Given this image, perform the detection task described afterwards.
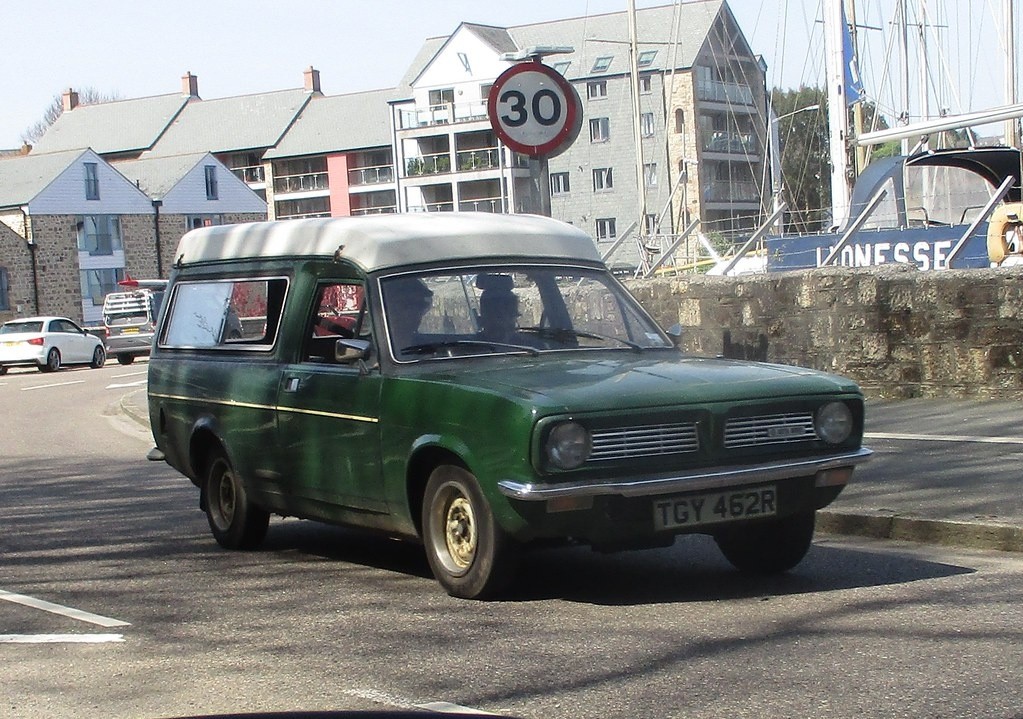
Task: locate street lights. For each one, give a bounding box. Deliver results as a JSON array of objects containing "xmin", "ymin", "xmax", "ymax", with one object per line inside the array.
[{"xmin": 769, "ymin": 105, "xmax": 819, "ymax": 190}]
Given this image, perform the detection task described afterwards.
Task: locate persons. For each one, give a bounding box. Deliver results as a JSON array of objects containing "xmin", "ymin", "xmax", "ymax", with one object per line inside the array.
[
  {"xmin": 382, "ymin": 278, "xmax": 433, "ymax": 348},
  {"xmin": 468, "ymin": 289, "xmax": 550, "ymax": 350}
]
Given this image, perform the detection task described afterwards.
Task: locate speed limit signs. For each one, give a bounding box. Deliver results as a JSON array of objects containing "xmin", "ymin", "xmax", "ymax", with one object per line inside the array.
[{"xmin": 488, "ymin": 62, "xmax": 576, "ymax": 156}]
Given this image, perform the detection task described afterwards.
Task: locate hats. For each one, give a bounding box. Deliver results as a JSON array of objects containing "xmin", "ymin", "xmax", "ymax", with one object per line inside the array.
[{"xmin": 385, "ymin": 277, "xmax": 433, "ymax": 298}]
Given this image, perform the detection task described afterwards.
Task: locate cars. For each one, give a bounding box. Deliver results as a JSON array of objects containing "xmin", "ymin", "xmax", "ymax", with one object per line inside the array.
[
  {"xmin": 148, "ymin": 211, "xmax": 874, "ymax": 601},
  {"xmin": 0, "ymin": 316, "xmax": 107, "ymax": 377}
]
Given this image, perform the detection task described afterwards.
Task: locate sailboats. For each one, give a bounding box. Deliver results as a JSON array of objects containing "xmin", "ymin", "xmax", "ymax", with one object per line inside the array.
[{"xmin": 584, "ymin": 0, "xmax": 1022, "ymax": 278}]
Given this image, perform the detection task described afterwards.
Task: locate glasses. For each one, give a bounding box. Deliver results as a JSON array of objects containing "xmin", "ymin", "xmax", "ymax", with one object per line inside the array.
[
  {"xmin": 490, "ymin": 312, "xmax": 522, "ymax": 320},
  {"xmin": 392, "ymin": 301, "xmax": 431, "ymax": 315}
]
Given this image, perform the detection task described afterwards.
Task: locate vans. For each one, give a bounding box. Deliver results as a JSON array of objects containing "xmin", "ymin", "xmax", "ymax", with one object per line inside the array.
[{"xmin": 102, "ymin": 289, "xmax": 244, "ymax": 365}]
